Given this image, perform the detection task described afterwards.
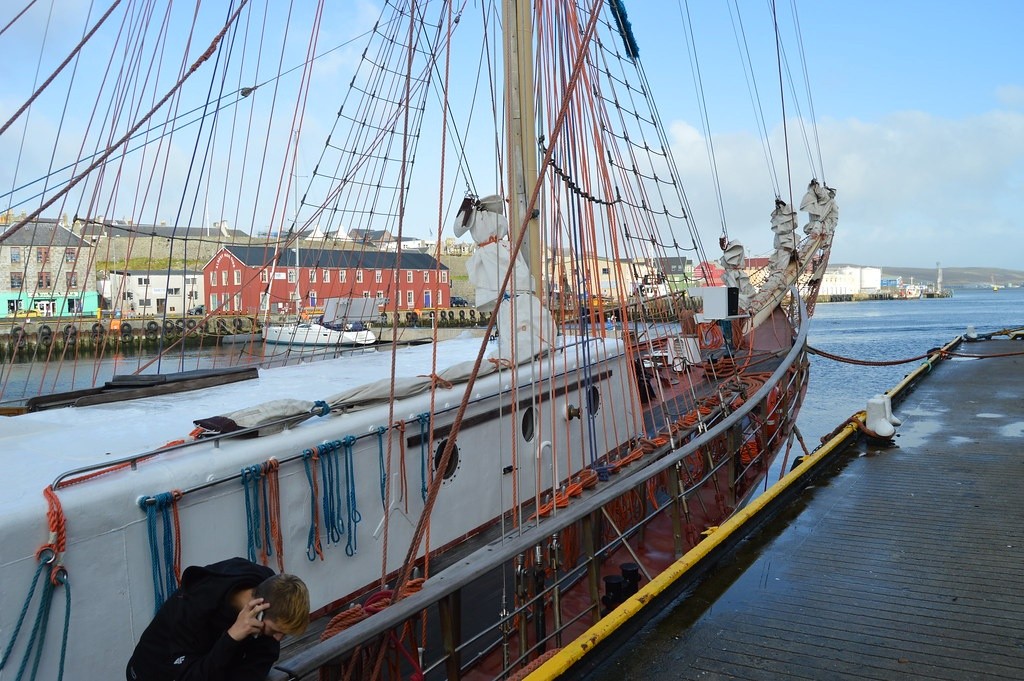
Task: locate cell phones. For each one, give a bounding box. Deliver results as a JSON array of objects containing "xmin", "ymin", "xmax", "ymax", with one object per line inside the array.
[{"xmin": 251, "ymin": 604, "xmax": 264, "ymax": 639}]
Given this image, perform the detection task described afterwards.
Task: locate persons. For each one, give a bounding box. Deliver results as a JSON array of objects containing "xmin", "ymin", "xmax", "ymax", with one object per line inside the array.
[{"xmin": 126, "ymin": 557, "xmax": 310, "ymax": 681}]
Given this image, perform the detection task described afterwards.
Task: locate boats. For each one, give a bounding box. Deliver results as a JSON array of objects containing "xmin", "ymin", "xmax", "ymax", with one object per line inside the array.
[
  {"xmin": 220, "ymin": 332, "xmax": 262, "ymax": 343},
  {"xmin": 629, "ymin": 240, "xmax": 673, "ymax": 321}
]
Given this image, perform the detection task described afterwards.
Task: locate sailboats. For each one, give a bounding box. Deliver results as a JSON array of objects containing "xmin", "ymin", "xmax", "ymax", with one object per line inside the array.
[
  {"xmin": 261, "ymin": 129, "xmax": 376, "ymax": 345},
  {"xmin": 0, "ymin": 1, "xmax": 838, "ymax": 681}
]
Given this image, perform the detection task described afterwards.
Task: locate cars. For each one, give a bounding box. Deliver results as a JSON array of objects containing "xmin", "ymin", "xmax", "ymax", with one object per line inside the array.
[
  {"xmin": 7, "ymin": 309, "xmax": 42, "ymax": 317},
  {"xmin": 450, "ymin": 297, "xmax": 468, "ymax": 307},
  {"xmin": 188, "ymin": 305, "xmax": 204, "ymax": 315}
]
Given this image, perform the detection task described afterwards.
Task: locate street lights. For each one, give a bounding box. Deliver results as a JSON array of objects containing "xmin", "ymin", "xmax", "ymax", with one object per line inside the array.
[{"xmin": 113, "ymin": 235, "xmax": 120, "ymax": 278}]
